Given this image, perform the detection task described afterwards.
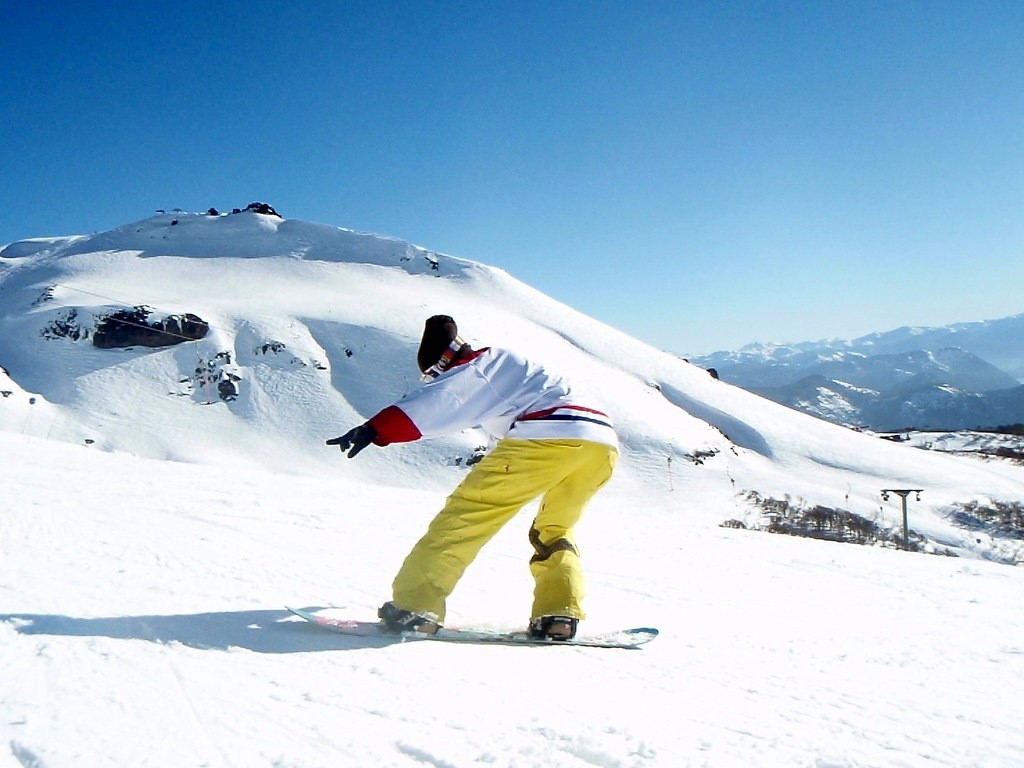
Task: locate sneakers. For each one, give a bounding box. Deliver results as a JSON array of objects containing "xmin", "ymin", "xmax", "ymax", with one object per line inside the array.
[
  {"xmin": 377, "ymin": 600, "xmax": 443, "ymax": 637},
  {"xmin": 526, "ymin": 614, "xmax": 579, "ymax": 640}
]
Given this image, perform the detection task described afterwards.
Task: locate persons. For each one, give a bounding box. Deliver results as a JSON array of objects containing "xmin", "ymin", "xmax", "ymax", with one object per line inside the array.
[{"xmin": 325, "ymin": 315, "xmax": 620, "ymax": 641}]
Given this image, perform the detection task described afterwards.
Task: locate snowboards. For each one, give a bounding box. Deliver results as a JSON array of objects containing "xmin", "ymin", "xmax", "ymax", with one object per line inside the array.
[{"xmin": 285, "ymin": 604, "xmax": 660, "ymax": 649}]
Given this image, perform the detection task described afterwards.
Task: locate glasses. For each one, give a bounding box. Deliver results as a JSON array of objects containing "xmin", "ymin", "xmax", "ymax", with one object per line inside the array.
[{"xmin": 419, "ymin": 364, "xmax": 445, "ymax": 383}]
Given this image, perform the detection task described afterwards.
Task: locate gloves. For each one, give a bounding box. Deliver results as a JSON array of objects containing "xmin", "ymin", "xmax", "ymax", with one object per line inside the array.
[{"xmin": 326, "ymin": 422, "xmax": 376, "ymax": 458}]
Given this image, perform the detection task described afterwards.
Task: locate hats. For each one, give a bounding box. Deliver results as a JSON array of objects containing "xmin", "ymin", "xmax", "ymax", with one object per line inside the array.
[{"xmin": 417, "ymin": 315, "xmax": 473, "ymax": 384}]
